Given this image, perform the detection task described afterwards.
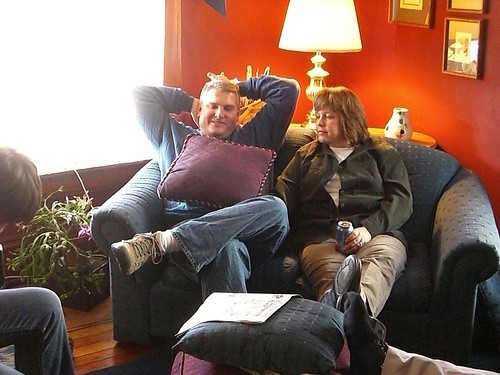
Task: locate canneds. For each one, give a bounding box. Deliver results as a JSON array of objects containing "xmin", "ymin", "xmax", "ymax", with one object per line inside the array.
[{"xmin": 335, "ymin": 221, "xmax": 354, "ymax": 254}]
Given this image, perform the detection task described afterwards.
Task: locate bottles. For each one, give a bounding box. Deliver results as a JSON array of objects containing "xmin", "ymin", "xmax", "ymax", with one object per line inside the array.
[{"xmin": 384, "ymin": 108, "xmax": 412, "ymax": 142}]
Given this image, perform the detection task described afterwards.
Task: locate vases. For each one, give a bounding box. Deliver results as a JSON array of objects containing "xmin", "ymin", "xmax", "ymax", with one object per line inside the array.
[{"xmin": 48, "ymin": 259, "xmax": 109, "ymax": 311}]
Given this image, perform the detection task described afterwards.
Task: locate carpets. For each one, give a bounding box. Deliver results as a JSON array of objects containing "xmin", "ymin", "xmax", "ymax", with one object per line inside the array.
[{"xmin": 82, "ymin": 342, "xmax": 176, "ymax": 375}]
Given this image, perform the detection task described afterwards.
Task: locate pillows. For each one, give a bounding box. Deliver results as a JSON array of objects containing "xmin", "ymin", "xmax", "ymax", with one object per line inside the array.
[
  {"xmin": 157, "ymin": 132, "xmax": 276, "ymax": 209},
  {"xmin": 170, "ymin": 298, "xmax": 346, "ymax": 375}
]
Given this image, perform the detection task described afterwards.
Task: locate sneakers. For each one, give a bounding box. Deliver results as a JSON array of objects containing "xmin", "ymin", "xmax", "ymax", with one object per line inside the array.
[{"xmin": 111, "ymin": 231, "xmax": 166, "ymax": 276}]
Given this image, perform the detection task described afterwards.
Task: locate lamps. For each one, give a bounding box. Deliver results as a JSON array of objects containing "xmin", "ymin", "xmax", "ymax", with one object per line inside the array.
[{"xmin": 277, "ymin": 0, "xmax": 363, "ymax": 129}]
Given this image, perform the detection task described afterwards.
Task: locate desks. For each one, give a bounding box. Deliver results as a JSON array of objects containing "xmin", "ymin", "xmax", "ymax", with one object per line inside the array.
[{"xmin": 288, "ymin": 122, "xmax": 438, "ymax": 148}]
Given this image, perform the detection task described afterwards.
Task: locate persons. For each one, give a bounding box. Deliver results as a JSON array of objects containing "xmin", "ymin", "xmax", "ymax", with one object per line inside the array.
[
  {"xmin": 111, "ymin": 75, "xmax": 301, "ymax": 303},
  {"xmin": 271, "ymin": 88, "xmax": 413, "ymax": 319},
  {"xmin": 0, "ymin": 148, "xmax": 75, "ymax": 375},
  {"xmin": 343, "ymin": 292, "xmax": 500, "ymax": 375}
]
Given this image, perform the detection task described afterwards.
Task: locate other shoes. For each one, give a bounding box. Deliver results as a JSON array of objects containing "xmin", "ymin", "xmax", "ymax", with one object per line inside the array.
[{"xmin": 344, "ymin": 292, "xmax": 389, "ymax": 375}]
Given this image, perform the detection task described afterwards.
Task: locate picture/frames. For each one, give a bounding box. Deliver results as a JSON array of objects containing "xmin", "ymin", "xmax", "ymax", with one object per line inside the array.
[
  {"xmin": 387, "ymin": 0, "xmax": 435, "ymax": 29},
  {"xmin": 441, "ymin": 17, "xmax": 485, "ymax": 79},
  {"xmin": 446, "ymin": 0, "xmax": 487, "ymax": 15}
]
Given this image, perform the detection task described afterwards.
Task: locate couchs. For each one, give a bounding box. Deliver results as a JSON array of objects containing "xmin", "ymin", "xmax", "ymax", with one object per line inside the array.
[{"xmin": 90, "ymin": 127, "xmax": 500, "ymax": 365}]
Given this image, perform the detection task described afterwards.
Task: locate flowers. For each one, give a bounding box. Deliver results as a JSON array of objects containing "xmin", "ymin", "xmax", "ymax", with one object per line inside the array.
[{"xmin": 6, "ymin": 184, "xmax": 106, "ymax": 300}]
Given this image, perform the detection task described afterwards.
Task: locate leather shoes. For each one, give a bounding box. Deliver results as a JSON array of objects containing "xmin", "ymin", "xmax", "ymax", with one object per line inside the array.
[{"xmin": 325, "ymin": 255, "xmax": 362, "ymax": 312}]
